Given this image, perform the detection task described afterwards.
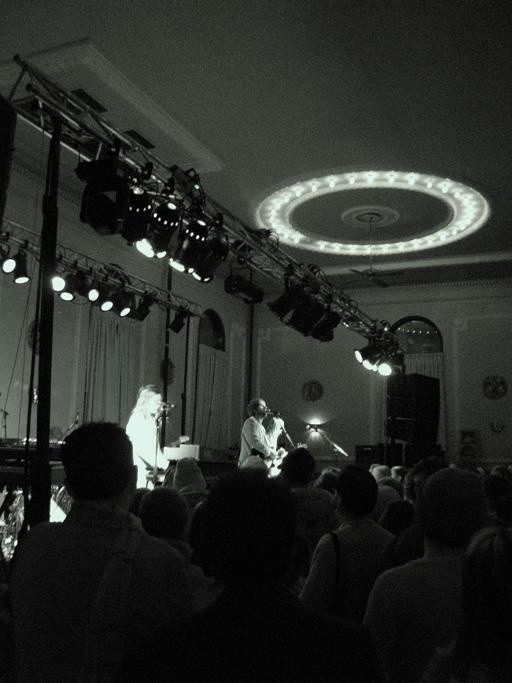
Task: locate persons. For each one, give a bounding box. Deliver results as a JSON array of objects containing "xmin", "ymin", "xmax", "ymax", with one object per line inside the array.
[{"xmin": 1, "ymin": 385, "xmax": 511, "ymax": 681}]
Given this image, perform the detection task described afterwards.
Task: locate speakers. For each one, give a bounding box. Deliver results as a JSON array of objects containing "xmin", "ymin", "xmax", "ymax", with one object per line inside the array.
[
  {"xmin": 384, "ymin": 373, "xmax": 440, "ymax": 444},
  {"xmin": 355, "ymin": 444, "xmax": 403, "ymax": 467},
  {"xmin": 404, "ymin": 445, "xmax": 441, "ymax": 467}
]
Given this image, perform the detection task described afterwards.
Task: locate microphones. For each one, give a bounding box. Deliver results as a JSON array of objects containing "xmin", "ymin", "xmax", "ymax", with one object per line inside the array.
[{"xmin": 265, "ymin": 409, "xmax": 281, "ymax": 416}]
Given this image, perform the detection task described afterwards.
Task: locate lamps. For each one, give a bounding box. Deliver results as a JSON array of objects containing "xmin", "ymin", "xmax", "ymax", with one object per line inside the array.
[{"xmin": 342, "ymin": 316, "xmax": 403, "ymax": 377}]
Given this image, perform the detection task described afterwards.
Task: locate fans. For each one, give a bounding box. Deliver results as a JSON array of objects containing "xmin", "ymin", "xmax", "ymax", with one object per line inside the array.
[{"xmin": 341, "ymin": 211, "xmax": 403, "ymax": 288}]
